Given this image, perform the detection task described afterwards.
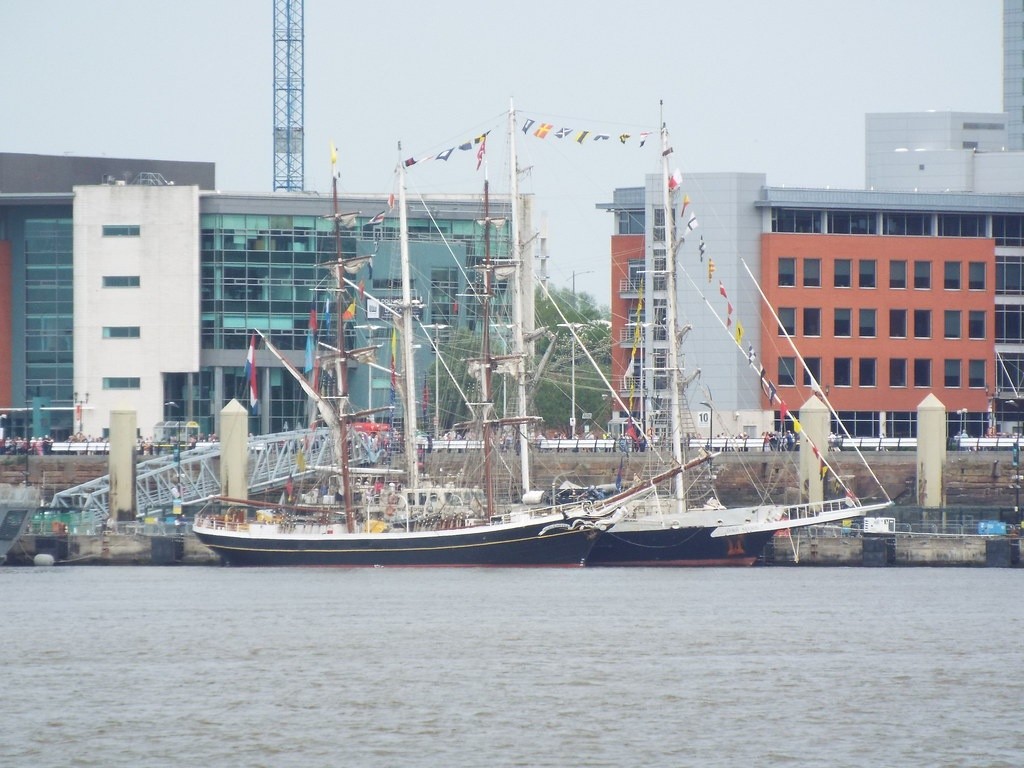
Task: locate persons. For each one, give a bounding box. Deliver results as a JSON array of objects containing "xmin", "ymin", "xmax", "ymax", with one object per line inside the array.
[
  {"xmin": 366, "ymin": 427, "xmax": 546, "ymax": 454},
  {"xmin": 4, "ymin": 433, "xmax": 54, "ymax": 456},
  {"xmin": 828, "ymin": 431, "xmax": 839, "ymax": 452},
  {"xmin": 553, "ymin": 431, "xmax": 617, "ymax": 453},
  {"xmin": 714, "ymin": 431, "xmax": 750, "ymax": 452},
  {"xmin": 374, "ymin": 480, "xmax": 382, "ymax": 495},
  {"xmin": 619, "ymin": 433, "xmax": 646, "ymax": 453},
  {"xmin": 956, "ymin": 428, "xmax": 972, "ymax": 452},
  {"xmin": 178, "ymin": 514, "xmax": 189, "ymax": 533},
  {"xmin": 363, "ymin": 479, "xmax": 370, "ymax": 486},
  {"xmin": 66, "ymin": 430, "xmax": 220, "ymax": 455},
  {"xmin": 248, "ymin": 432, "xmax": 255, "ymax": 455},
  {"xmin": 354, "ymin": 478, "xmax": 361, "ymax": 486},
  {"xmin": 761, "ymin": 430, "xmax": 796, "ymax": 452}
]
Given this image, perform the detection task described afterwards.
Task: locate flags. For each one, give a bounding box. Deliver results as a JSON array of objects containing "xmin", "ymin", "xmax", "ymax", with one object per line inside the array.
[
  {"xmin": 244, "ymin": 332, "xmax": 259, "ymax": 416},
  {"xmin": 284, "ymin": 417, "xmax": 319, "ymax": 502},
  {"xmin": 519, "ymin": 116, "xmax": 651, "ymax": 151},
  {"xmin": 662, "ymin": 144, "xmax": 850, "ymax": 497},
  {"xmin": 302, "ymin": 129, "xmax": 491, "ymax": 394}
]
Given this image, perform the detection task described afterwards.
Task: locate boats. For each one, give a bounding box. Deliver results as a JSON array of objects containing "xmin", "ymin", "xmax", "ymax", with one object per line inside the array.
[
  {"xmin": 575, "ymin": 94, "xmax": 901, "ymax": 567},
  {"xmin": 192, "ymin": 94, "xmax": 722, "ymax": 571}
]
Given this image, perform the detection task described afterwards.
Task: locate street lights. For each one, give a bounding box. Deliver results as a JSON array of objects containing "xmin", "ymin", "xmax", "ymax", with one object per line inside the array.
[
  {"xmin": 700, "ymin": 401, "xmax": 712, "ymax": 452},
  {"xmin": 962, "ymin": 408, "xmax": 968, "ymax": 434},
  {"xmin": 1005, "ymin": 400, "xmax": 1022, "ymax": 526},
  {"xmin": 73, "ymin": 392, "xmax": 90, "ymax": 432}
]
